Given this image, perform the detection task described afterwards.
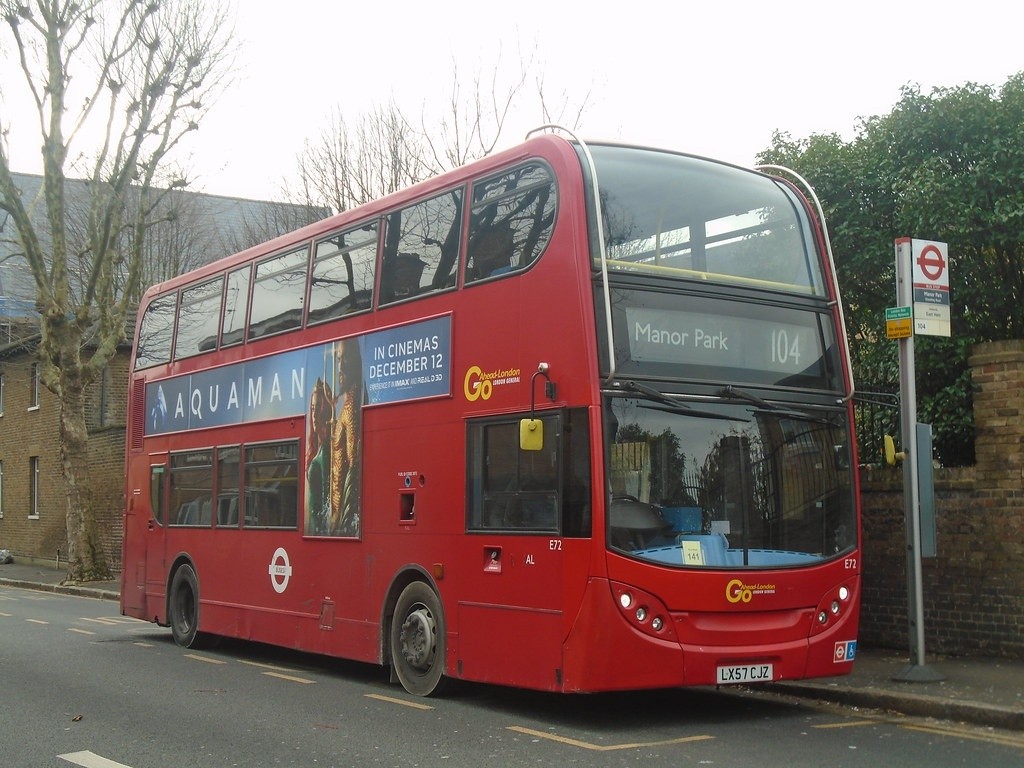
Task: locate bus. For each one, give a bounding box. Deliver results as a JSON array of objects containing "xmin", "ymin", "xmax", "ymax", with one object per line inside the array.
[{"xmin": 119, "ymin": 124, "xmax": 905, "ymax": 697}]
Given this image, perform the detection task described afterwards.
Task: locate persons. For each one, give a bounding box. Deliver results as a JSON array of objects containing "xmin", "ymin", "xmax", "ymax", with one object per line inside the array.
[
  {"xmin": 304, "ymin": 335, "xmax": 369, "ymax": 537},
  {"xmin": 489, "ymin": 439, "xmax": 587, "ymax": 538}
]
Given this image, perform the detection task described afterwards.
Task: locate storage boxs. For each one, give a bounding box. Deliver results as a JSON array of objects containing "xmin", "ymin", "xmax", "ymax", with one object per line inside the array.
[{"xmin": 661, "ymin": 506, "xmax": 702, "ymax": 533}]
